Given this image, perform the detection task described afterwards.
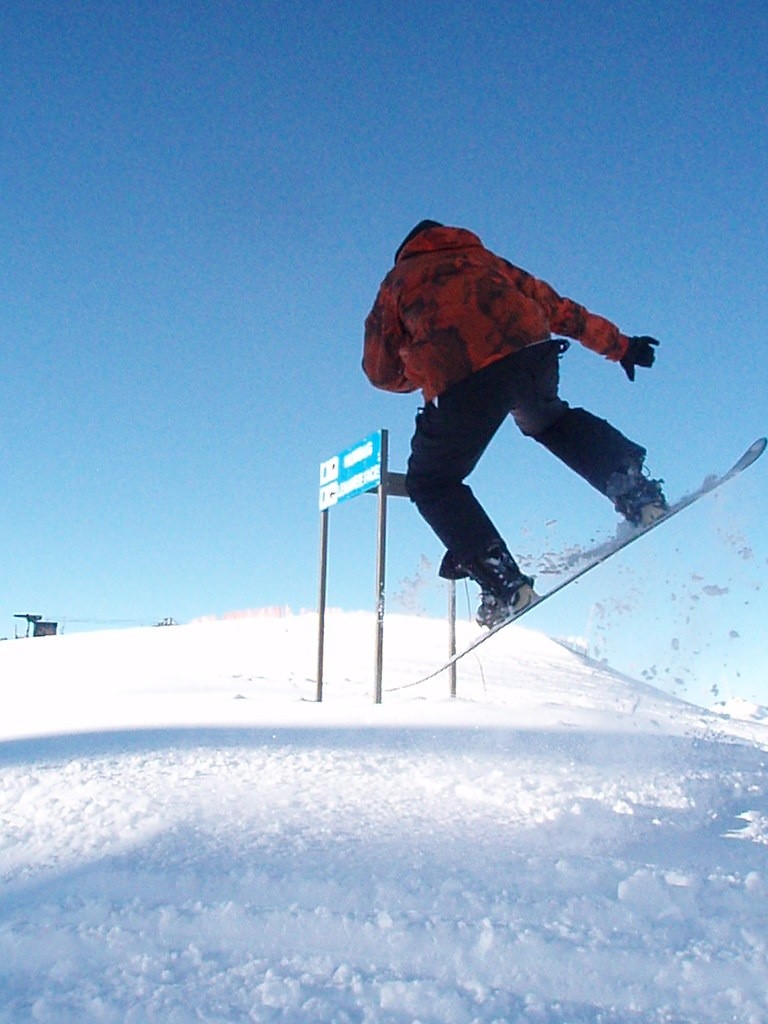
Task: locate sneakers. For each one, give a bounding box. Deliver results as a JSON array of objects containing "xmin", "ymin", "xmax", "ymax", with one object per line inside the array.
[
  {"xmin": 615, "ymin": 462, "xmax": 670, "ymax": 528},
  {"xmin": 475, "ymin": 570, "xmax": 540, "ymax": 626}
]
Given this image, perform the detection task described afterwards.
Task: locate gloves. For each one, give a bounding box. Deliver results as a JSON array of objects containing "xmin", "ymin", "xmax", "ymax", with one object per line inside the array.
[{"xmin": 620, "ymin": 336, "xmax": 661, "ymax": 382}]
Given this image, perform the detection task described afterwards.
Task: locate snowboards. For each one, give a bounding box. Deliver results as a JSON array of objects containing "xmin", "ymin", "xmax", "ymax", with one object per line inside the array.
[{"xmin": 376, "ymin": 433, "xmax": 768, "ymax": 697}]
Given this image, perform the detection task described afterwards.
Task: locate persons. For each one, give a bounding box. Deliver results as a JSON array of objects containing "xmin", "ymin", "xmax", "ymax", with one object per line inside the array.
[{"xmin": 361, "ymin": 216, "xmax": 672, "ymax": 633}]
